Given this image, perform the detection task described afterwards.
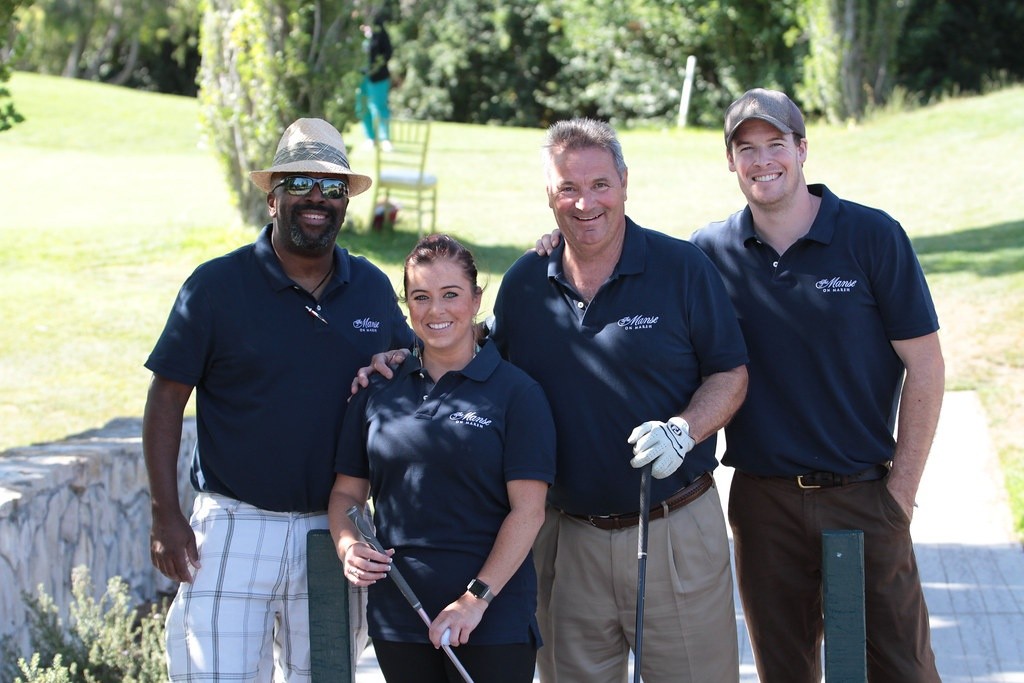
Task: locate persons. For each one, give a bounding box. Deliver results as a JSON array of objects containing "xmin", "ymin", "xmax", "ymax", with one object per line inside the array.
[
  {"xmin": 142, "ymin": 118, "xmax": 496, "ymax": 683},
  {"xmin": 353, "ymin": 17, "xmax": 394, "ymax": 152},
  {"xmin": 328, "ymin": 233, "xmax": 556, "ymax": 683},
  {"xmin": 524, "ymin": 88, "xmax": 943, "ymax": 683},
  {"xmin": 348, "ymin": 116, "xmax": 749, "ymax": 683}
]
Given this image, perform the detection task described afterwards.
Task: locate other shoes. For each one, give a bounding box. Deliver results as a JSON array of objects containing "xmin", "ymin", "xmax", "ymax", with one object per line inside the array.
[
  {"xmin": 361, "ymin": 140, "xmax": 373, "ymax": 152},
  {"xmin": 379, "ymin": 141, "xmax": 391, "ymax": 153}
]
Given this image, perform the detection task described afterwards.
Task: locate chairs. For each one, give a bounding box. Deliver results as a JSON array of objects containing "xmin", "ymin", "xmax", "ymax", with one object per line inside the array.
[{"xmin": 369, "ymin": 114, "xmax": 439, "ymax": 241}]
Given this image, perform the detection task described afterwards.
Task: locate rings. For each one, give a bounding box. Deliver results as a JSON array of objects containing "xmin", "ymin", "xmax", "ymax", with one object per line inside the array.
[{"xmin": 350, "ymin": 567, "xmax": 358, "ymax": 573}]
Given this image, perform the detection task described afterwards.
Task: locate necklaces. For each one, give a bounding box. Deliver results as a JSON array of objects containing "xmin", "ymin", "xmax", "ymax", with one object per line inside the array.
[{"xmin": 310, "ymin": 260, "xmax": 334, "ymax": 294}]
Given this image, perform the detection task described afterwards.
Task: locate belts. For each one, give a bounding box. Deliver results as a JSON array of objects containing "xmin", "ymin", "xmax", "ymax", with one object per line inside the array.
[
  {"xmin": 779, "ymin": 464, "xmax": 889, "ymax": 488},
  {"xmin": 554, "ymin": 472, "xmax": 713, "ymax": 529}
]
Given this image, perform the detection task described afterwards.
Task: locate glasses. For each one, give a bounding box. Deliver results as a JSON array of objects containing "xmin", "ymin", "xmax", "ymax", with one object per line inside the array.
[{"xmin": 271, "ymin": 175, "xmax": 350, "ymax": 199}]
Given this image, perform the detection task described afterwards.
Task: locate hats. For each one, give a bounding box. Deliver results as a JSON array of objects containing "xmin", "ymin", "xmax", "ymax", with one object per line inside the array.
[
  {"xmin": 724, "ymin": 88, "xmax": 805, "ymax": 147},
  {"xmin": 248, "ymin": 118, "xmax": 372, "ymax": 198}
]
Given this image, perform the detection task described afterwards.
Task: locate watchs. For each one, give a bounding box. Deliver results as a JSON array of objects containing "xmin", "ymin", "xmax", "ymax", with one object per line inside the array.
[{"xmin": 467, "ymin": 578, "xmax": 495, "ymax": 604}]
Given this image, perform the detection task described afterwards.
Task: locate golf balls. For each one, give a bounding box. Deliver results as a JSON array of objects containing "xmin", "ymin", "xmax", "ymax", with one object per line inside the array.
[{"xmin": 440, "ymin": 628, "xmax": 452, "ymax": 645}]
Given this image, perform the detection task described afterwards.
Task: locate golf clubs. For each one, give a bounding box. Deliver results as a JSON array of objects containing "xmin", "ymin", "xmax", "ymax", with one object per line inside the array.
[
  {"xmin": 632, "ymin": 462, "xmax": 653, "ymax": 683},
  {"xmin": 345, "ymin": 504, "xmax": 473, "ymax": 683}
]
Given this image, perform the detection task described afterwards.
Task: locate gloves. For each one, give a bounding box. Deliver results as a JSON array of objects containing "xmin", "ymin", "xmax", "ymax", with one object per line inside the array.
[{"xmin": 628, "ymin": 417, "xmax": 695, "ymax": 479}]
